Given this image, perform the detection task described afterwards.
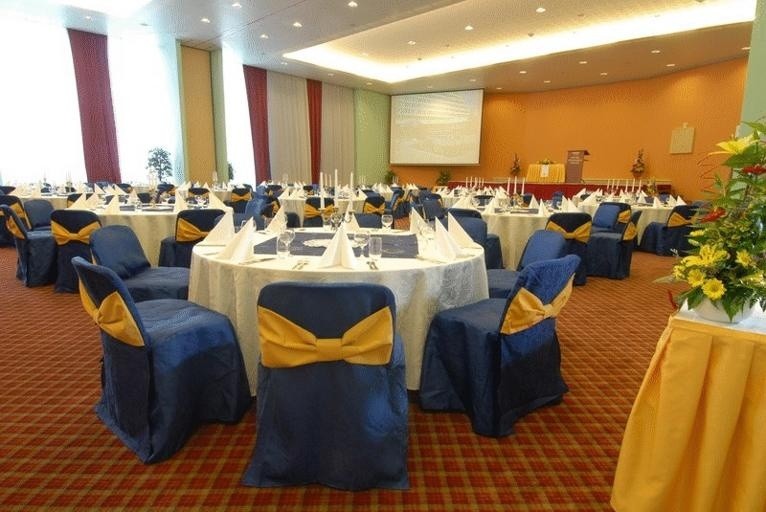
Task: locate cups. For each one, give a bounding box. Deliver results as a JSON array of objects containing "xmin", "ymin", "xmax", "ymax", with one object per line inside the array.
[
  {"xmin": 264, "ymin": 217, "xmax": 273, "ymax": 231},
  {"xmin": 275, "ymin": 235, "xmax": 289, "ymax": 261},
  {"xmin": 134, "ymin": 201, "xmax": 141, "ymax": 213},
  {"xmin": 268, "ymin": 179, "xmax": 273, "ymax": 187},
  {"xmin": 368, "ymin": 237, "xmax": 382, "ymax": 267}
]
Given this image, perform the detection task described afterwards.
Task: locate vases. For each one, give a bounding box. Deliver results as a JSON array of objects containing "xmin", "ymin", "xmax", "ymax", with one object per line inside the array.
[
  {"xmin": 633, "ymin": 171, "xmax": 642, "ymax": 179},
  {"xmin": 690, "ymin": 294, "xmax": 754, "ymax": 324}
]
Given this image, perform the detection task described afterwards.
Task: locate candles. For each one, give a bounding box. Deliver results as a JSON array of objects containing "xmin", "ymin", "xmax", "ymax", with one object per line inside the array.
[
  {"xmin": 605, "ymin": 178, "xmax": 612, "ymax": 194},
  {"xmin": 520, "ymin": 177, "xmax": 526, "ymax": 196},
  {"xmin": 631, "ymin": 179, "xmax": 636, "ymax": 196},
  {"xmin": 334, "ymin": 168, "xmax": 341, "ymax": 208},
  {"xmin": 465, "ymin": 175, "xmax": 486, "ymax": 190},
  {"xmin": 513, "ymin": 175, "xmax": 517, "ymax": 196},
  {"xmin": 319, "ymin": 172, "xmax": 326, "ymax": 210},
  {"xmin": 624, "ymin": 178, "xmax": 629, "ymax": 196},
  {"xmin": 350, "ymin": 172, "xmax": 355, "ymax": 210},
  {"xmin": 638, "ymin": 177, "xmax": 642, "ymax": 193},
  {"xmin": 614, "ymin": 179, "xmax": 621, "ymax": 197},
  {"xmin": 505, "ymin": 178, "xmax": 511, "ymax": 196}
]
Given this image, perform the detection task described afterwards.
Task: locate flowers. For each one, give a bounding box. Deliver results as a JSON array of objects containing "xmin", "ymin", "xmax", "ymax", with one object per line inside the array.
[
  {"xmin": 670, "ymin": 117, "xmax": 766, "ymax": 319},
  {"xmin": 632, "ymin": 149, "xmax": 646, "ymax": 173}
]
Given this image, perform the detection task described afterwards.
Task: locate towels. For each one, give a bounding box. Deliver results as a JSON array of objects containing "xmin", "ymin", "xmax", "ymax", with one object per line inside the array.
[
  {"xmin": 277, "ymin": 182, "xmax": 280, "ymax": 185},
  {"xmin": 226, "ymin": 183, "xmax": 232, "ymax": 191},
  {"xmin": 343, "ymin": 183, "xmax": 349, "ymax": 191},
  {"xmin": 597, "ymin": 189, "xmax": 603, "ymax": 197},
  {"xmin": 620, "ymin": 190, "xmax": 623, "ymax": 197},
  {"xmin": 298, "ymin": 187, "xmax": 305, "ymax": 196},
  {"xmin": 477, "ymin": 188, "xmax": 482, "ymax": 195},
  {"xmin": 676, "ymin": 196, "xmax": 686, "ymax": 206},
  {"xmin": 265, "ymin": 205, "xmax": 289, "ymax": 234},
  {"xmin": 269, "ymin": 180, "xmax": 274, "ymax": 185},
  {"xmin": 302, "ymin": 181, "xmax": 306, "ymax": 186},
  {"xmin": 127, "ymin": 188, "xmax": 139, "ymax": 203},
  {"xmin": 31, "ymin": 183, "xmax": 42, "ymax": 199},
  {"xmin": 406, "ymin": 183, "xmax": 409, "ymax": 190},
  {"xmin": 595, "ymin": 188, "xmax": 599, "ymax": 193},
  {"xmin": 450, "ymin": 185, "xmax": 459, "ymax": 196},
  {"xmin": 76, "ymin": 181, "xmax": 85, "ymax": 193},
  {"xmin": 94, "ymin": 183, "xmax": 104, "ymax": 194},
  {"xmin": 208, "ymin": 192, "xmax": 225, "ymax": 209},
  {"xmin": 620, "ymin": 194, "xmax": 628, "ymax": 202},
  {"xmin": 627, "ymin": 192, "xmax": 631, "ymax": 201},
  {"xmin": 413, "ymin": 183, "xmax": 418, "ymax": 189},
  {"xmin": 386, "ymin": 185, "xmax": 392, "ymax": 192},
  {"xmin": 584, "ymin": 192, "xmax": 596, "ymax": 206},
  {"xmin": 451, "ymin": 196, "xmax": 464, "ymax": 208},
  {"xmin": 237, "ymin": 184, "xmax": 244, "ymax": 188},
  {"xmin": 106, "ymin": 184, "xmax": 115, "ymax": 195},
  {"xmin": 434, "ymin": 216, "xmax": 465, "ymax": 260},
  {"xmin": 440, "ymin": 187, "xmax": 449, "ymax": 196},
  {"xmin": 203, "ymin": 182, "xmax": 209, "ymax": 190},
  {"xmin": 539, "ymin": 199, "xmax": 550, "ymax": 217},
  {"xmin": 316, "ymin": 222, "xmax": 360, "ymax": 271},
  {"xmin": 103, "ymin": 194, "xmax": 121, "ymax": 214},
  {"xmin": 668, "ymin": 195, "xmax": 676, "ymax": 207},
  {"xmin": 372, "ymin": 182, "xmax": 378, "ymax": 190},
  {"xmin": 379, "ymin": 184, "xmax": 384, "ymax": 193},
  {"xmin": 483, "ymin": 198, "xmax": 499, "ymax": 213},
  {"xmin": 68, "ymin": 190, "xmax": 86, "ymax": 210},
  {"xmin": 222, "ymin": 182, "xmax": 227, "ymax": 190},
  {"xmin": 500, "ymin": 186, "xmax": 506, "ymax": 192},
  {"xmin": 214, "ymin": 216, "xmax": 255, "ymax": 262},
  {"xmin": 180, "ymin": 181, "xmax": 185, "ymax": 189},
  {"xmin": 642, "ymin": 191, "xmax": 648, "ymax": 197},
  {"xmin": 561, "ymin": 195, "xmax": 568, "ymax": 212},
  {"xmin": 294, "ymin": 181, "xmax": 299, "ymax": 187},
  {"xmin": 359, "ymin": 189, "xmax": 366, "ymax": 199},
  {"xmin": 280, "ymin": 187, "xmax": 289, "ymax": 198},
  {"xmin": 184, "ymin": 181, "xmax": 192, "ymax": 188},
  {"xmin": 409, "ymin": 207, "xmax": 436, "ymax": 242},
  {"xmin": 577, "ymin": 188, "xmax": 586, "ymax": 197},
  {"xmin": 459, "ymin": 195, "xmax": 477, "ymax": 210},
  {"xmin": 87, "ymin": 193, "xmax": 99, "ymax": 209},
  {"xmin": 653, "ymin": 197, "xmax": 662, "ymax": 208},
  {"xmin": 489, "ymin": 186, "xmax": 493, "ymax": 194},
  {"xmin": 260, "ymin": 180, "xmax": 267, "ymax": 188},
  {"xmin": 199, "ymin": 210, "xmax": 236, "ymax": 246},
  {"xmin": 9, "ymin": 183, "xmax": 25, "ymax": 196},
  {"xmin": 458, "ymin": 186, "xmax": 463, "ymax": 192},
  {"xmin": 483, "ymin": 187, "xmax": 491, "ymax": 195},
  {"xmin": 638, "ymin": 192, "xmax": 646, "ymax": 203},
  {"xmin": 409, "ymin": 183, "xmax": 413, "ymax": 189},
  {"xmin": 568, "ymin": 198, "xmax": 580, "ymax": 212},
  {"xmin": 291, "ymin": 188, "xmax": 298, "ymax": 199},
  {"xmin": 341, "ymin": 201, "xmax": 361, "ymax": 231},
  {"xmin": 529, "ymin": 194, "xmax": 540, "ymax": 208},
  {"xmin": 298, "ymin": 181, "xmax": 302, "ymax": 187},
  {"xmin": 436, "ymin": 188, "xmax": 441, "ymax": 194},
  {"xmin": 447, "ymin": 212, "xmax": 480, "ymax": 249},
  {"xmin": 195, "ymin": 180, "xmax": 200, "ymax": 188},
  {"xmin": 607, "ymin": 192, "xmax": 614, "ymax": 202},
  {"xmin": 113, "ymin": 184, "xmax": 125, "ymax": 194}
]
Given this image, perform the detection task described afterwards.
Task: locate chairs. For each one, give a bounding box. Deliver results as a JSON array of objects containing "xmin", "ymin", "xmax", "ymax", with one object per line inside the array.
[
  {"xmin": 303, "ymin": 197, "xmax": 334, "ymax": 226},
  {"xmin": 548, "ymin": 213, "xmax": 591, "ymax": 286},
  {"xmin": 590, "ymin": 202, "xmax": 632, "ymax": 233},
  {"xmin": 226, "ymin": 187, "xmax": 251, "ymax": 214},
  {"xmin": 23, "ymin": 199, "xmax": 52, "ymax": 229},
  {"xmin": 362, "ymin": 195, "xmax": 387, "ymax": 214},
  {"xmin": 590, "ymin": 219, "xmax": 636, "ymax": 280},
  {"xmin": 242, "ymin": 281, "xmax": 405, "ymax": 490},
  {"xmin": 641, "ymin": 206, "xmax": 697, "ymax": 255},
  {"xmin": 157, "ymin": 184, "xmax": 173, "ymax": 203},
  {"xmin": 187, "ymin": 186, "xmax": 211, "ymax": 207},
  {"xmin": 450, "ymin": 207, "xmax": 503, "ymax": 268},
  {"xmin": 50, "ymin": 208, "xmax": 102, "ymax": 294},
  {"xmin": 5, "ymin": 193, "xmax": 32, "ymax": 229},
  {"xmin": 0, "ymin": 205, "xmax": 55, "ymax": 285},
  {"xmin": 88, "ymin": 223, "xmax": 190, "ymax": 299},
  {"xmin": 425, "ymin": 254, "xmax": 581, "ymax": 440},
  {"xmin": 71, "ymin": 255, "xmax": 250, "ymax": 466},
  {"xmin": 159, "ymin": 209, "xmax": 225, "ymax": 268},
  {"xmin": 486, "ymin": 231, "xmax": 567, "ymax": 295}
]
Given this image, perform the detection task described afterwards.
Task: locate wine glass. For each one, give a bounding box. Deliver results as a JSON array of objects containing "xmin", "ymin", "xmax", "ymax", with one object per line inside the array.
[
  {"xmin": 557, "ymin": 201, "xmax": 562, "ymax": 211},
  {"xmin": 198, "ymin": 198, "xmax": 207, "ymax": 209},
  {"xmin": 212, "ymin": 171, "xmax": 218, "ymax": 189},
  {"xmin": 547, "ymin": 200, "xmax": 553, "ymax": 211},
  {"xmin": 282, "ymin": 173, "xmax": 289, "ymax": 187},
  {"xmin": 354, "ymin": 230, "xmax": 370, "ymax": 262},
  {"xmin": 392, "ymin": 176, "xmax": 398, "ymax": 187},
  {"xmin": 417, "ymin": 219, "xmax": 429, "ymax": 232},
  {"xmin": 241, "ymin": 221, "xmax": 256, "ymax": 234},
  {"xmin": 133, "ymin": 197, "xmax": 141, "ymax": 210},
  {"xmin": 381, "ymin": 214, "xmax": 394, "ymax": 234},
  {"xmin": 164, "ymin": 194, "xmax": 170, "ymax": 205},
  {"xmin": 194, "ymin": 194, "xmax": 202, "ymax": 207},
  {"xmin": 331, "ymin": 213, "xmax": 342, "ymax": 232},
  {"xmin": 517, "ymin": 197, "xmax": 523, "ymax": 212},
  {"xmin": 276, "ymin": 229, "xmax": 295, "ymax": 258}
]
